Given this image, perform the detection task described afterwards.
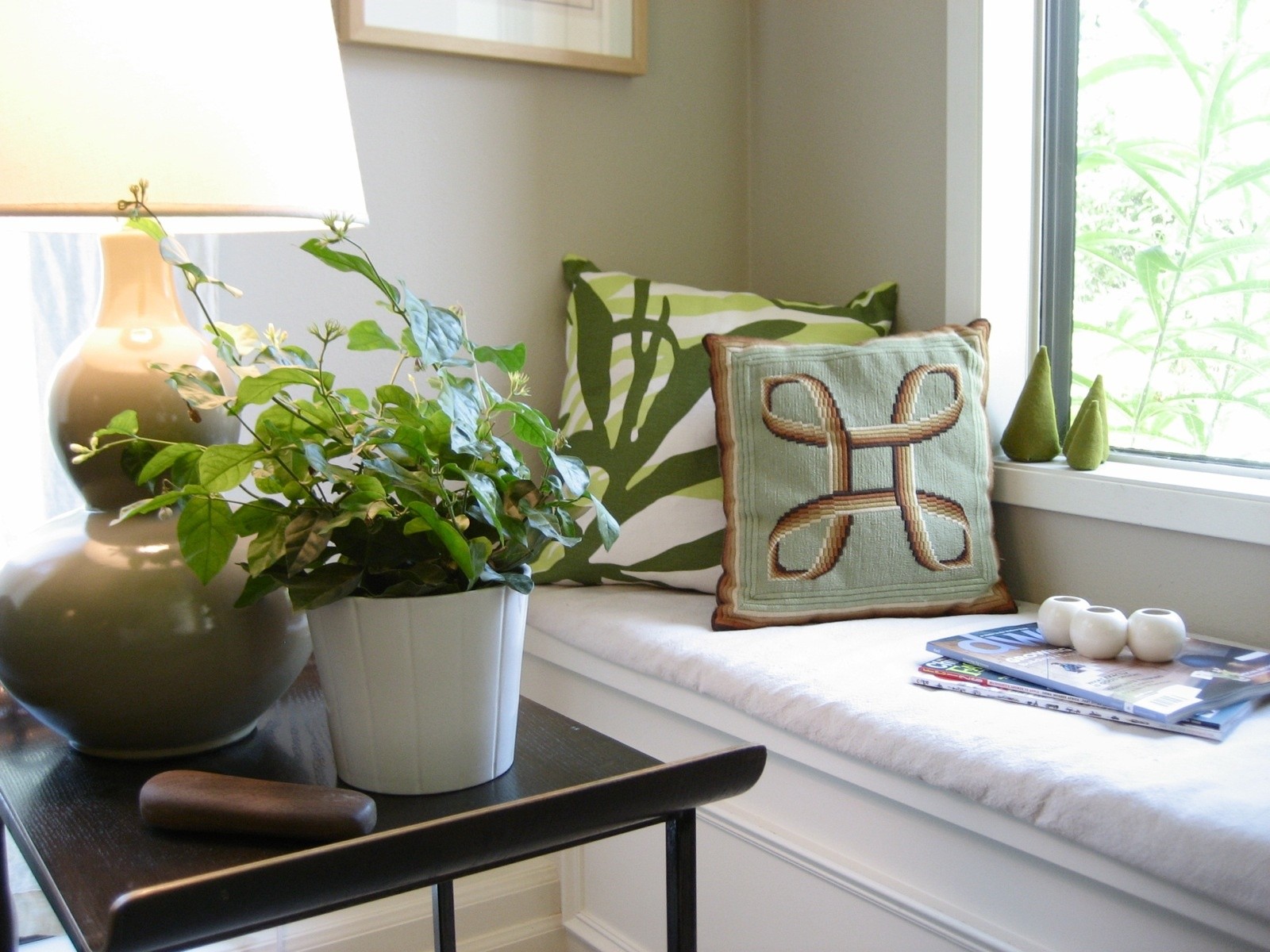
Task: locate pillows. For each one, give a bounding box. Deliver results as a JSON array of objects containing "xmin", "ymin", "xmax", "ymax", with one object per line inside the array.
[
  {"xmin": 703, "ymin": 315, "xmax": 1017, "ymax": 631},
  {"xmin": 530, "ymin": 257, "xmax": 899, "ymax": 586}
]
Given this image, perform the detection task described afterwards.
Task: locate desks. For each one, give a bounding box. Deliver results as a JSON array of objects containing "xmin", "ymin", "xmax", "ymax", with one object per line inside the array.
[{"xmin": 0, "ymin": 656, "xmax": 768, "ymax": 952}]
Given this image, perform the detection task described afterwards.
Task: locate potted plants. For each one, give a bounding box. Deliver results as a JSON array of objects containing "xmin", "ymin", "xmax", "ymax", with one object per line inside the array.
[{"xmin": 94, "ymin": 186, "xmax": 623, "ymax": 800}]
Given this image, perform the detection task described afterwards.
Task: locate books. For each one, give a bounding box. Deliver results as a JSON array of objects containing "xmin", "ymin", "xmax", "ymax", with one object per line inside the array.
[{"xmin": 909, "ymin": 621, "xmax": 1270, "ymax": 742}]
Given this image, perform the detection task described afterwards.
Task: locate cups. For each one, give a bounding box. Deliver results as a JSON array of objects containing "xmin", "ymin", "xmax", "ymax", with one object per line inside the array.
[
  {"xmin": 1036, "ymin": 594, "xmax": 1091, "ymax": 648},
  {"xmin": 1070, "ymin": 605, "xmax": 1127, "ymax": 659},
  {"xmin": 1125, "ymin": 607, "xmax": 1186, "ymax": 662}
]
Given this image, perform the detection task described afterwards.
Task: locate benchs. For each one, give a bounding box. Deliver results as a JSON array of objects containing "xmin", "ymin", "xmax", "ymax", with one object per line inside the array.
[{"xmin": 522, "ymin": 595, "xmax": 1270, "ymax": 952}]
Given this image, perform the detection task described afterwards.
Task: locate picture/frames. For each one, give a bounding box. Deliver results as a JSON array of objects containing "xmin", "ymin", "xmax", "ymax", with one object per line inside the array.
[{"xmin": 338, "ymin": 0, "xmax": 648, "ymax": 78}]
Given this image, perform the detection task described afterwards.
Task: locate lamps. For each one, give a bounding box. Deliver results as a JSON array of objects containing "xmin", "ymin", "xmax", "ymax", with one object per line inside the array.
[{"xmin": 0, "ymin": 0, "xmax": 367, "ymax": 754}]
